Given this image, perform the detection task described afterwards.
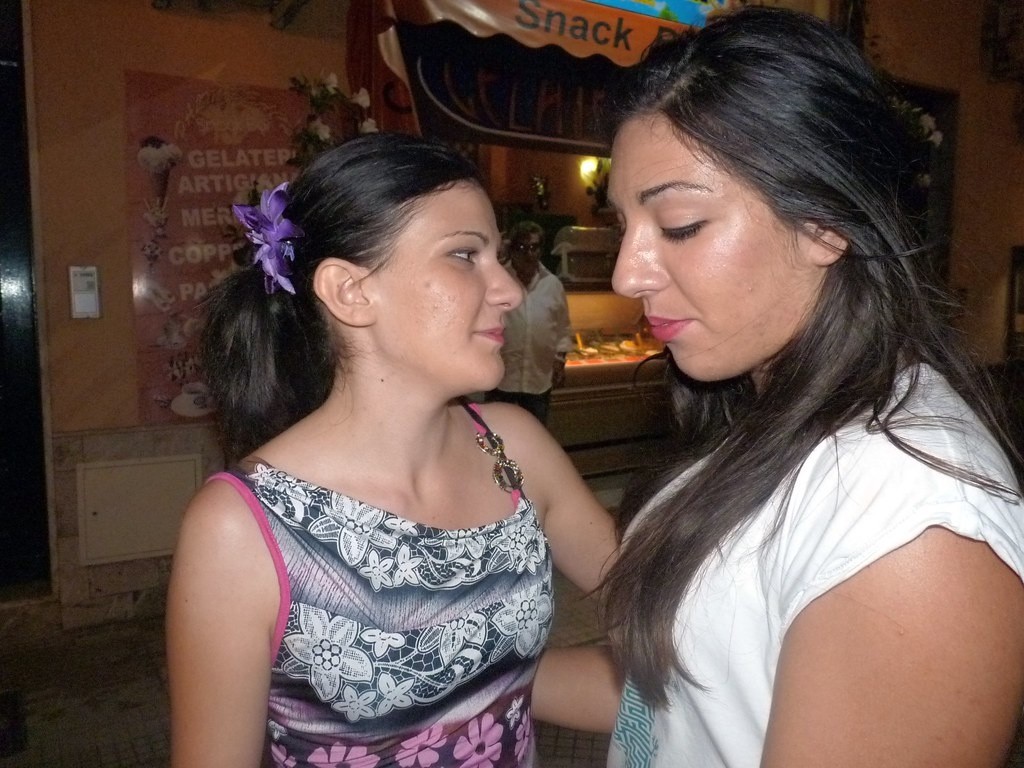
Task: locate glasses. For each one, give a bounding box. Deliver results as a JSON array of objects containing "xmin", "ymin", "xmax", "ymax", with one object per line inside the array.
[{"xmin": 509, "ymin": 242, "xmax": 541, "ymax": 253}]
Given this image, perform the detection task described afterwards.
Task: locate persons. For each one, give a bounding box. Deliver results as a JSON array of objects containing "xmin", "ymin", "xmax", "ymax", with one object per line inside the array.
[
  {"xmin": 530, "ymin": 7, "xmax": 1024, "ymax": 768},
  {"xmin": 165, "ymin": 134, "xmax": 626, "ymax": 768},
  {"xmin": 485, "ymin": 220, "xmax": 572, "ymax": 428}
]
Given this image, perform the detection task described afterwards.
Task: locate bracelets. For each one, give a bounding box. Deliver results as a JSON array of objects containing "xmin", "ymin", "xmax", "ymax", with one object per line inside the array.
[{"xmin": 555, "ymin": 354, "xmax": 566, "ymax": 363}]
